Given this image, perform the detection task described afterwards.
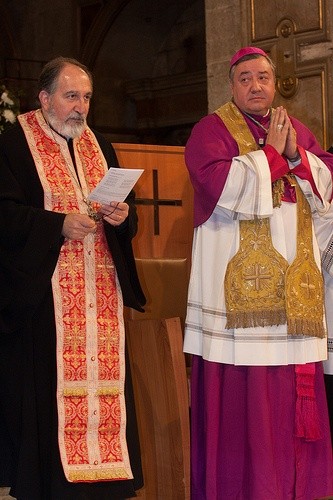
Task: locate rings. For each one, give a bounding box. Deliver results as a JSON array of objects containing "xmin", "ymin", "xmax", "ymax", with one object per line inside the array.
[{"xmin": 276, "ymin": 123, "xmax": 283, "ymax": 128}]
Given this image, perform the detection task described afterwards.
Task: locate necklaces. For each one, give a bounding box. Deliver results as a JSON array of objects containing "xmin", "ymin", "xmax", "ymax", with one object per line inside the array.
[
  {"xmin": 243, "ymin": 111, "xmax": 270, "ymax": 134},
  {"xmin": 45, "ymin": 117, "xmax": 104, "ymax": 223}
]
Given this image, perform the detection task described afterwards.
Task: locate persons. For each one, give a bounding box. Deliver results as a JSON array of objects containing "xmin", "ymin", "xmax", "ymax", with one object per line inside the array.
[
  {"xmin": 184, "ymin": 47, "xmax": 333, "ymax": 500},
  {"xmin": 0, "ymin": 60, "xmax": 146, "ymax": 500}
]
